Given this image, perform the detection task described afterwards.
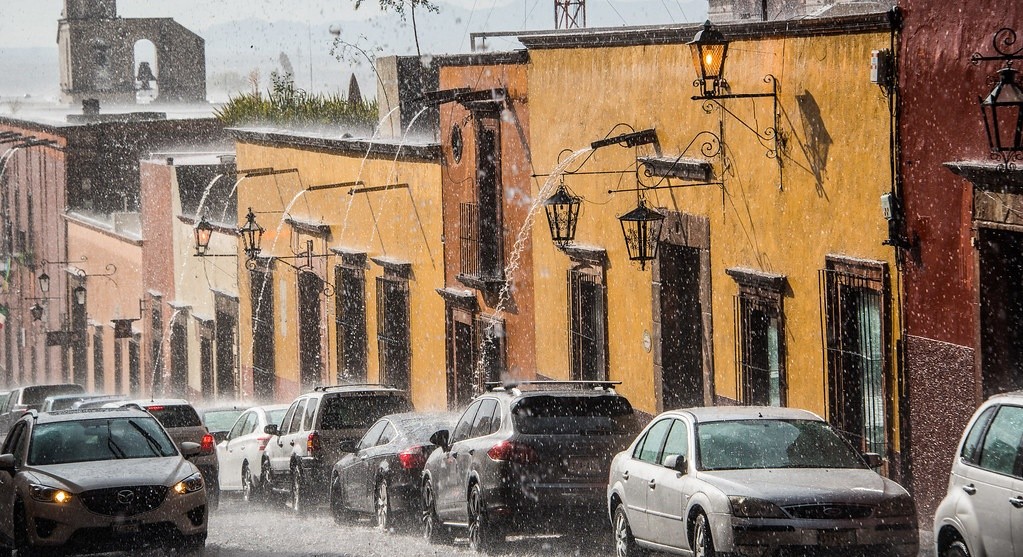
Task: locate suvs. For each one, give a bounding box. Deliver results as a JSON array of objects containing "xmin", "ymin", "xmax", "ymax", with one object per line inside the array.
[
  {"xmin": 260, "ymin": 382, "xmax": 415, "ymax": 516},
  {"xmin": 421, "ymin": 380, "xmax": 643, "ymax": 554}
]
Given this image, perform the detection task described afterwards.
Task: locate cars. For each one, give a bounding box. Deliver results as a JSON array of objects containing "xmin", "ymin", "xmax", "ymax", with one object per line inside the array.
[
  {"xmin": 218, "ymin": 404, "xmax": 291, "ymax": 502},
  {"xmin": 328, "ymin": 410, "xmax": 462, "ymax": 531},
  {"xmin": 932, "ymin": 390, "xmax": 1023, "ymax": 556},
  {"xmin": 607, "ymin": 406, "xmax": 920, "ymax": 557},
  {"xmin": 0, "ymin": 382, "xmax": 249, "ymax": 511},
  {"xmin": 0, "ymin": 404, "xmax": 208, "ymax": 557}
]
